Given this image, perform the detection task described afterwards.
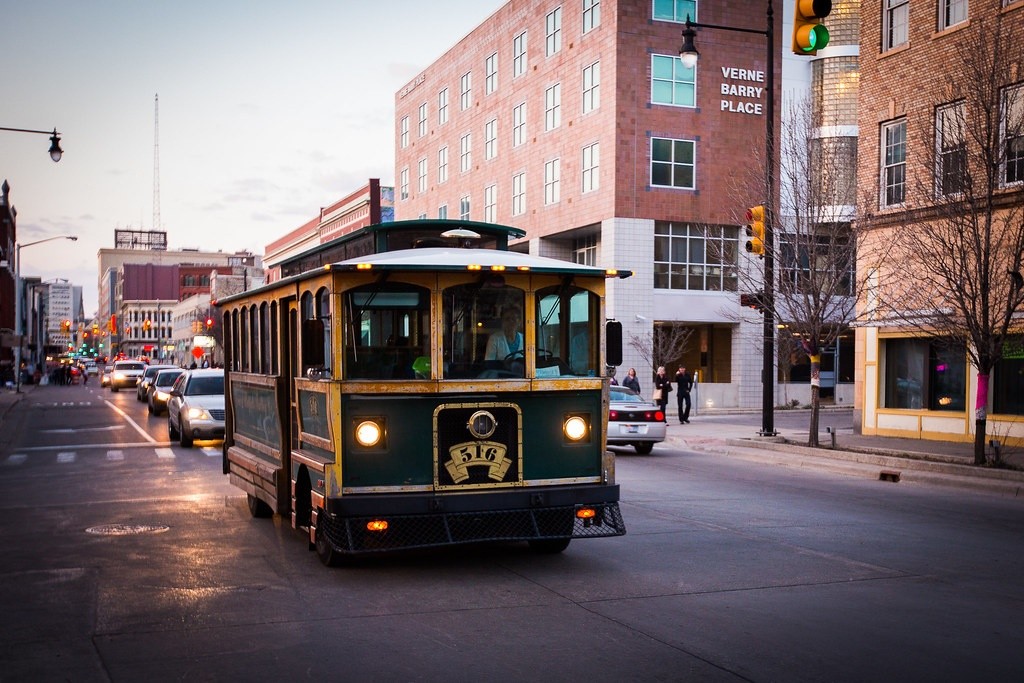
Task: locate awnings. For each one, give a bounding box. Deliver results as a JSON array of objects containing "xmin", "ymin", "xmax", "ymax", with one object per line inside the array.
[{"xmin": 849, "ymin": 306, "xmax": 955, "ymax": 327}]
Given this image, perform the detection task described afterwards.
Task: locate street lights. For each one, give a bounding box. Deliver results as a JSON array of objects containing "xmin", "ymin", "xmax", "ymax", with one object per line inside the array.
[
  {"xmin": 678, "ymin": 15, "xmax": 774, "ymax": 437},
  {"xmin": 17, "ymin": 236, "xmax": 78, "ymax": 394}
]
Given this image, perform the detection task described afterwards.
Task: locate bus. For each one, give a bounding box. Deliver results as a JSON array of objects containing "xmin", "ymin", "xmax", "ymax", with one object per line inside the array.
[{"xmin": 211, "ymin": 218, "xmax": 627, "ymax": 569}]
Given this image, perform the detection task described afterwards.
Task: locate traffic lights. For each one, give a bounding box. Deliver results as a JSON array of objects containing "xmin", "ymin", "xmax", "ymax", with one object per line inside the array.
[
  {"xmin": 746, "ymin": 205, "xmax": 765, "ymax": 256},
  {"xmin": 792, "ymin": 1, "xmax": 831, "ymax": 56},
  {"xmin": 107, "ymin": 321, "xmax": 111, "ymax": 331},
  {"xmin": 207, "ymin": 320, "xmax": 212, "ymax": 330},
  {"xmin": 749, "ymin": 295, "xmax": 763, "ymax": 308},
  {"xmin": 127, "ymin": 328, "xmax": 130, "ymax": 335},
  {"xmin": 65, "ymin": 321, "xmax": 70, "ymax": 332},
  {"xmin": 144, "ymin": 320, "xmax": 150, "ymax": 329},
  {"xmin": 192, "ymin": 322, "xmax": 203, "ymax": 333}
]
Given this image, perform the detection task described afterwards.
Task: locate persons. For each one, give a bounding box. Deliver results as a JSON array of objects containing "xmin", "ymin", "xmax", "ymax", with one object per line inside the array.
[
  {"xmin": 655, "ymin": 366, "xmax": 673, "ymax": 426},
  {"xmin": 675, "ymin": 364, "xmax": 692, "ymax": 424},
  {"xmin": 622, "ymin": 368, "xmax": 640, "ymax": 395},
  {"xmin": 27, "ymin": 361, "xmax": 89, "ymax": 386},
  {"xmin": 484, "ymin": 307, "xmax": 524, "ymax": 360},
  {"xmin": 162, "ymin": 351, "xmax": 224, "ymax": 369}
]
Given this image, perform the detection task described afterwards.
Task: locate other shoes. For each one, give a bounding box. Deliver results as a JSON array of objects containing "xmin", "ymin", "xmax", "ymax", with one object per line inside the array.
[
  {"xmin": 665, "ymin": 423, "xmax": 669, "ymax": 426},
  {"xmin": 680, "ymin": 420, "xmax": 690, "ymax": 424}
]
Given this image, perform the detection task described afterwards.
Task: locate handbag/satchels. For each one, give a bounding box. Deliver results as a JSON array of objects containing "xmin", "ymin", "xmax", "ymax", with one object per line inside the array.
[{"xmin": 653, "ymin": 388, "xmax": 663, "ymax": 400}]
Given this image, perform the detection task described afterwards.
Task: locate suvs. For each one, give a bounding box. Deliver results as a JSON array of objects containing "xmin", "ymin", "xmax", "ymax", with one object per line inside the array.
[
  {"xmin": 136, "ymin": 355, "xmax": 149, "ymax": 364},
  {"xmin": 167, "ymin": 369, "xmax": 229, "ymax": 448},
  {"xmin": 111, "ymin": 361, "xmax": 146, "ymax": 392}
]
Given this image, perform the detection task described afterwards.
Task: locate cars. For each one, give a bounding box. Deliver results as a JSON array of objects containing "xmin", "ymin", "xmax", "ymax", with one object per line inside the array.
[
  {"xmin": 113, "ymin": 353, "xmax": 129, "ymax": 362},
  {"xmin": 606, "ymin": 383, "xmax": 667, "ymax": 455},
  {"xmin": 94, "ymin": 355, "xmax": 108, "ymax": 364},
  {"xmin": 136, "ymin": 365, "xmax": 177, "ymax": 402},
  {"xmin": 47, "ymin": 356, "xmax": 98, "ymax": 385},
  {"xmin": 101, "ymin": 366, "xmax": 114, "ymax": 386},
  {"xmin": 146, "ymin": 369, "xmax": 185, "ymax": 416}
]
